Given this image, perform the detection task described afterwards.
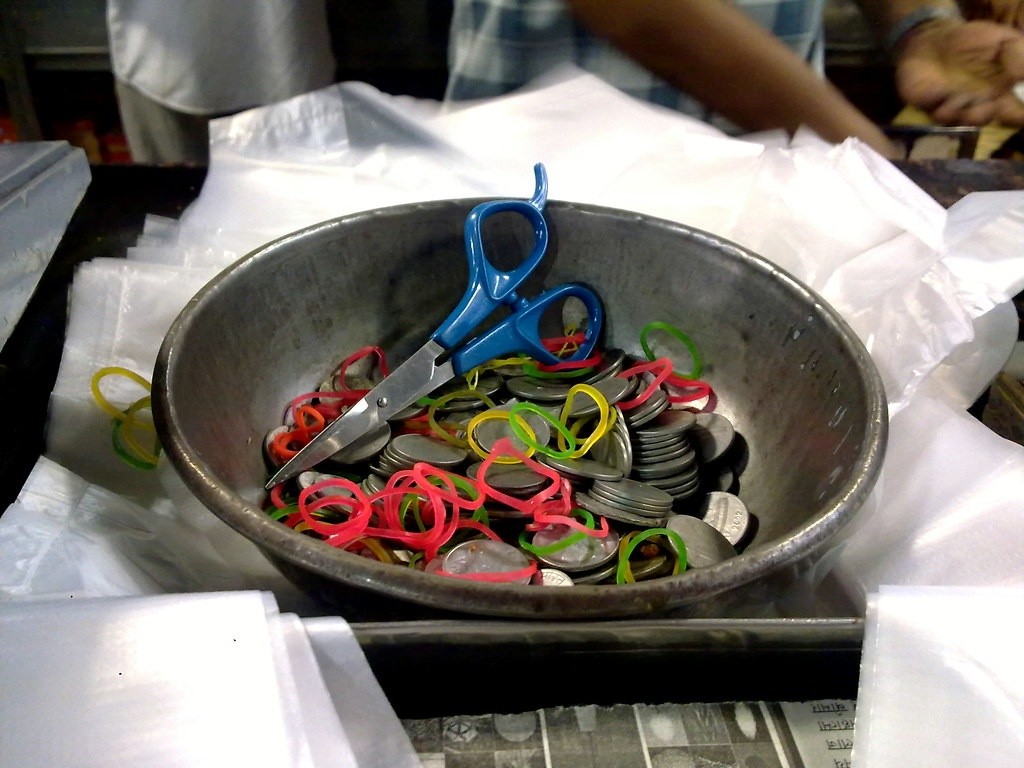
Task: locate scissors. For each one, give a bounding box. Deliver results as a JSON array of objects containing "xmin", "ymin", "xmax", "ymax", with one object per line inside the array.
[{"xmin": 262, "ymin": 160, "xmax": 607, "ymax": 491}]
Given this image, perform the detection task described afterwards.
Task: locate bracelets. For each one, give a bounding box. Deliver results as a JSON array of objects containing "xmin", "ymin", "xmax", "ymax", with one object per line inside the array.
[{"xmin": 878, "ymin": 5, "xmax": 963, "ymax": 55}]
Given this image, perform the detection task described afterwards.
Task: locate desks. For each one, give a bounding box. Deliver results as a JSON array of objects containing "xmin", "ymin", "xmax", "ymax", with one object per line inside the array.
[{"xmin": 0, "ymin": 158, "xmax": 1023, "ymax": 767}]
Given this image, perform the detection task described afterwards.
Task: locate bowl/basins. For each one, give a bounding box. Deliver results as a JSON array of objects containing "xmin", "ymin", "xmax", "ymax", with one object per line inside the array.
[{"xmin": 153, "ymin": 194, "xmax": 891, "ymax": 617}]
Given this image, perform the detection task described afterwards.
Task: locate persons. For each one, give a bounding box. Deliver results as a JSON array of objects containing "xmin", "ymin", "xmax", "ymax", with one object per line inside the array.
[
  {"xmin": 103, "ymin": 0, "xmax": 338, "ymax": 168},
  {"xmin": 439, "ymin": 0, "xmax": 1024, "ymax": 159}
]
